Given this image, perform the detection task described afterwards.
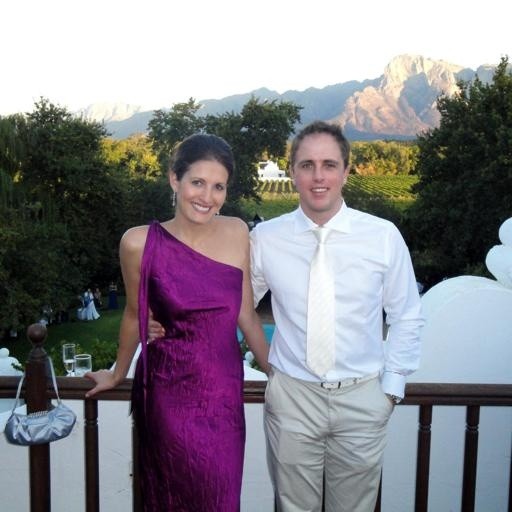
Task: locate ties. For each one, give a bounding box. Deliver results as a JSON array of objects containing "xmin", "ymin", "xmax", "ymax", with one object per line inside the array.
[{"xmin": 304, "ymin": 227, "xmax": 337, "ymax": 380}]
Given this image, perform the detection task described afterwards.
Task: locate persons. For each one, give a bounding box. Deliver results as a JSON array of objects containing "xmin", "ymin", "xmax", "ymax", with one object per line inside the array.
[
  {"xmin": 83, "ymin": 132, "xmax": 272, "ymax": 512},
  {"xmin": 84, "ymin": 288, "xmax": 100, "ymax": 321},
  {"xmin": 94, "ymin": 287, "xmax": 104, "ymax": 309},
  {"xmin": 147, "ymin": 119, "xmax": 425, "ymax": 512},
  {"xmin": 108, "ymin": 281, "xmax": 121, "ymax": 309}
]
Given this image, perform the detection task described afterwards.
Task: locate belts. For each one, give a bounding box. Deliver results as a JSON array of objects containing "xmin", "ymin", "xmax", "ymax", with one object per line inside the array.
[{"xmin": 275, "ymin": 366, "xmax": 381, "ymax": 391}]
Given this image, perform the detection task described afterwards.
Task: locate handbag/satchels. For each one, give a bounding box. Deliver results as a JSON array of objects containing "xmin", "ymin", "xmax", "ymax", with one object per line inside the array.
[{"xmin": 3, "ymin": 400, "xmax": 79, "ymax": 448}]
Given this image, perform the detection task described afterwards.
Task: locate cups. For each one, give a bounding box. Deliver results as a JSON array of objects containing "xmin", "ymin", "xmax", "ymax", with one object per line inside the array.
[{"xmin": 62, "ymin": 343, "xmax": 92, "ymax": 378}]
